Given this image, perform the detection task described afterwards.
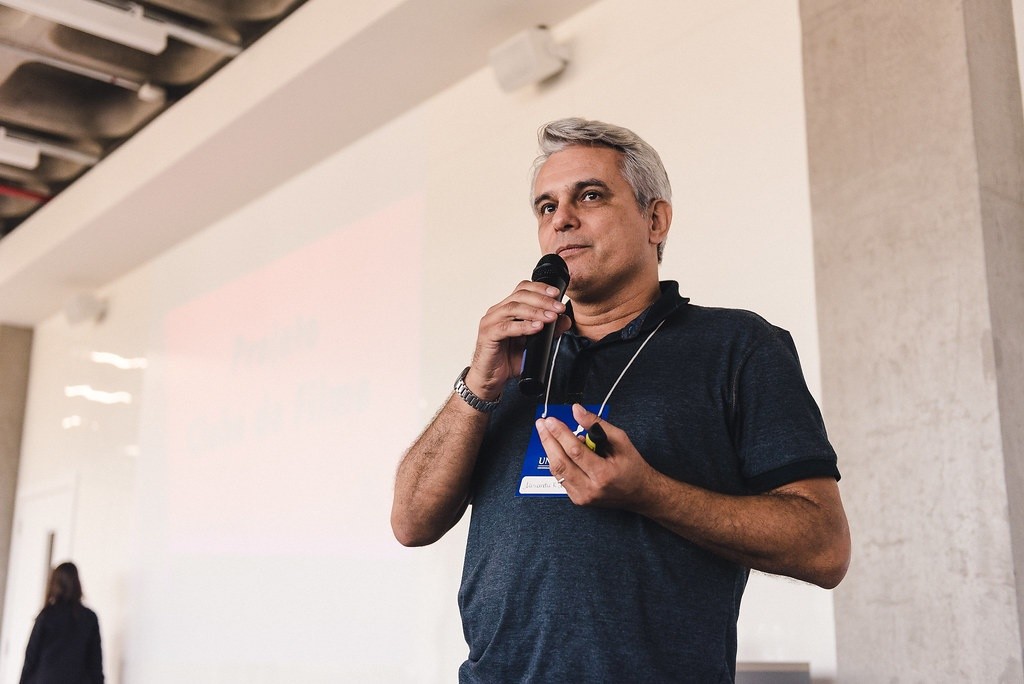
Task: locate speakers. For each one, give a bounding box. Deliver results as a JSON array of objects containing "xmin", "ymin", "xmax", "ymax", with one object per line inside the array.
[{"xmin": 491, "ymin": 22, "xmax": 564, "ymax": 91}]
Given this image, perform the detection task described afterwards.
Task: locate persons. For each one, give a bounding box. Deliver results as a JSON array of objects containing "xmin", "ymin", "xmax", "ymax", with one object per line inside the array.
[
  {"xmin": 390, "ymin": 116, "xmax": 851, "ymax": 684},
  {"xmin": 20, "ymin": 563, "xmax": 105, "ymax": 684}
]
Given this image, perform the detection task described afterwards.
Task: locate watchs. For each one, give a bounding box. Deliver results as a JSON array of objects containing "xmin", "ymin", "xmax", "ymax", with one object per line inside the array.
[{"xmin": 454, "ymin": 367, "xmax": 502, "ymax": 413}]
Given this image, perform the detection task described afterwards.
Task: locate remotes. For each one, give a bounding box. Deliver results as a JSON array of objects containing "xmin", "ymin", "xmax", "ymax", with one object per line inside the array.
[{"xmin": 585, "ymin": 422, "xmax": 608, "ymax": 458}]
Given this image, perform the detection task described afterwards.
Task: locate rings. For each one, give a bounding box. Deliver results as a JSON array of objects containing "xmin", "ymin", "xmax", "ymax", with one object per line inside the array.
[{"xmin": 557, "ymin": 478, "xmax": 565, "ymax": 484}]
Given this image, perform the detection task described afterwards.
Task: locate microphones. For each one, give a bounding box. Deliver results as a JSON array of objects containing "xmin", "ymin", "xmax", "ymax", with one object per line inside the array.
[{"xmin": 518, "ymin": 254, "xmax": 570, "ymax": 398}]
